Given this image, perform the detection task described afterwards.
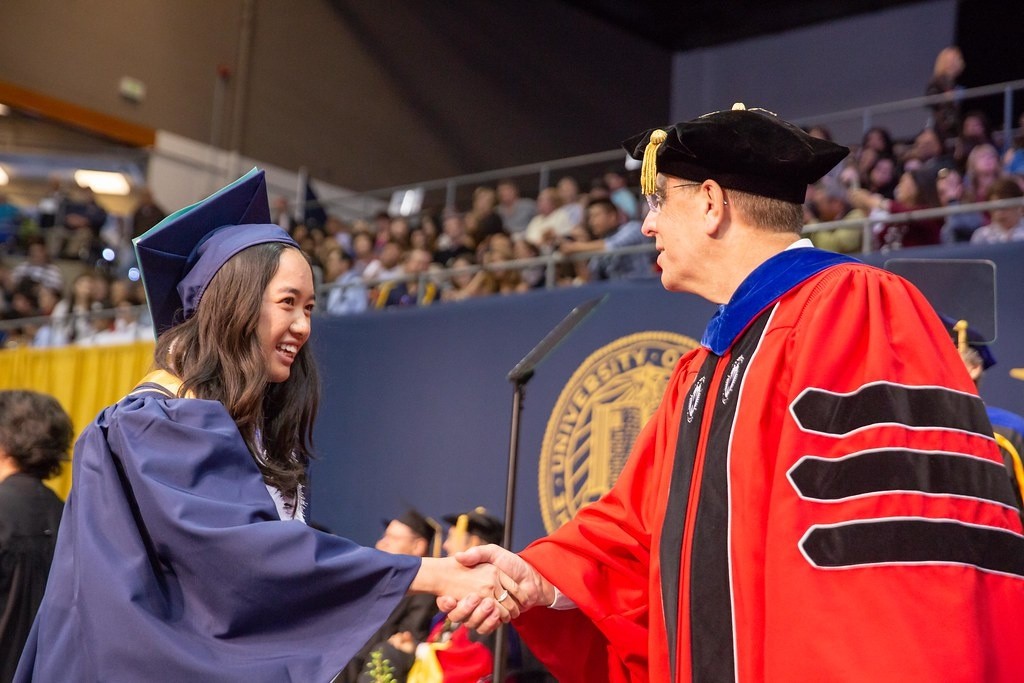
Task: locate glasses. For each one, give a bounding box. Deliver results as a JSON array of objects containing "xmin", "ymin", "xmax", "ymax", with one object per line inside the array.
[{"xmin": 645, "ymin": 183, "xmax": 727, "ymax": 211}]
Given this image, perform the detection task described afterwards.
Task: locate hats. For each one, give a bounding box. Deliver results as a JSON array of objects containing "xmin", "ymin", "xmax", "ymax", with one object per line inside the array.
[
  {"xmin": 619, "ymin": 101, "xmax": 852, "ymax": 206},
  {"xmin": 440, "ymin": 512, "xmax": 503, "ymax": 544},
  {"xmin": 384, "ymin": 509, "xmax": 436, "ymax": 542},
  {"xmin": 938, "ymin": 314, "xmax": 997, "ymax": 371},
  {"xmin": 132, "ymin": 167, "xmax": 301, "ymax": 348}
]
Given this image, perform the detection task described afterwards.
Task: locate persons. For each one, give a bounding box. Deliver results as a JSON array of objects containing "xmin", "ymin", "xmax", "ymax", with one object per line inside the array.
[{"xmin": 0, "ymin": 50, "xmax": 1024, "ymax": 683}]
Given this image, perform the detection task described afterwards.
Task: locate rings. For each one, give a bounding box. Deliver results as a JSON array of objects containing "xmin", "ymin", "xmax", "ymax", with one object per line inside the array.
[{"xmin": 497, "ymin": 590, "xmax": 508, "ymax": 602}]
